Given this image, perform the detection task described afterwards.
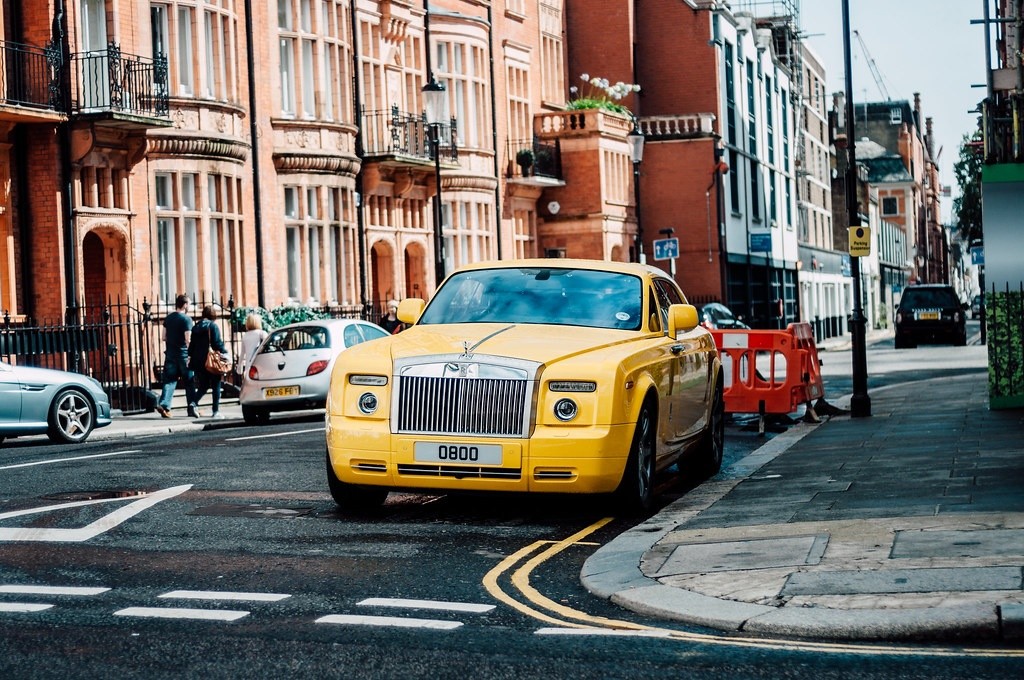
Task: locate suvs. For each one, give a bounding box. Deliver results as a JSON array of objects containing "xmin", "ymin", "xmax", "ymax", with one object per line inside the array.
[{"xmin": 892, "ymin": 283, "xmax": 969, "ymax": 349}]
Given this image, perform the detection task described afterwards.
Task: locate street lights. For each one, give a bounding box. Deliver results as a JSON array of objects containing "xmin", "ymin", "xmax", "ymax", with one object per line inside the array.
[
  {"xmin": 422, "ymin": 71, "xmax": 452, "ymax": 291},
  {"xmin": 626, "ymin": 121, "xmax": 645, "ymax": 264}
]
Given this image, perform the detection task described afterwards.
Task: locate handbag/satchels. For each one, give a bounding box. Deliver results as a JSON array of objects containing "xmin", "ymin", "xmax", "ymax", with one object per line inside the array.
[{"xmin": 204, "ymin": 348, "xmax": 229, "ymax": 376}]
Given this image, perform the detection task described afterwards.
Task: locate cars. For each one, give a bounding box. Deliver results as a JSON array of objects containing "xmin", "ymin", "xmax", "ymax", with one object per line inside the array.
[
  {"xmin": 696, "ymin": 301, "xmax": 753, "ymax": 331},
  {"xmin": 324, "ymin": 257, "xmax": 725, "ymax": 513},
  {"xmin": 971, "ymin": 295, "xmax": 987, "ymax": 319},
  {"xmin": 0, "ymin": 360, "xmax": 111, "ymax": 444},
  {"xmin": 239, "ymin": 319, "xmax": 394, "ymax": 426}
]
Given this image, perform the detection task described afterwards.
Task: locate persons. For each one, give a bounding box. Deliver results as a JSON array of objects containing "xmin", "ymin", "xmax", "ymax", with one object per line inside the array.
[
  {"xmin": 379, "ymin": 299, "xmax": 406, "ymax": 335},
  {"xmin": 236, "ymin": 314, "xmax": 275, "ymax": 378},
  {"xmin": 186, "ymin": 306, "xmax": 228, "ymax": 419},
  {"xmin": 154, "ymin": 294, "xmax": 200, "ymax": 418}
]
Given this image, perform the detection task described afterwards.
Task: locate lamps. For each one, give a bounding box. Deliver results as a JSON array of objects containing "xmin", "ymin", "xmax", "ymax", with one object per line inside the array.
[{"xmin": 707, "ymin": 160, "xmax": 729, "ymax": 191}]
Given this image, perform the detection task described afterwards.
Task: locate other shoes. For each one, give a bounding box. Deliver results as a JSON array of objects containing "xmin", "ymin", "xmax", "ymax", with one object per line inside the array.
[
  {"xmin": 187, "ymin": 403, "xmax": 221, "ymax": 418},
  {"xmin": 156, "ymin": 406, "xmax": 170, "ymax": 419}
]
